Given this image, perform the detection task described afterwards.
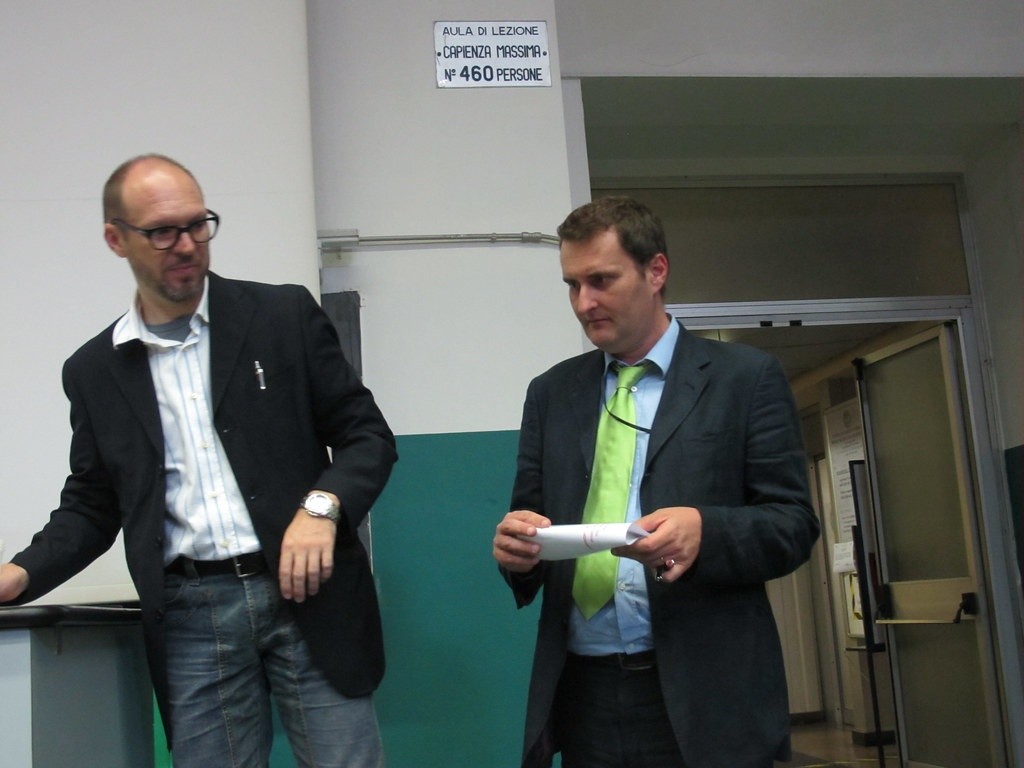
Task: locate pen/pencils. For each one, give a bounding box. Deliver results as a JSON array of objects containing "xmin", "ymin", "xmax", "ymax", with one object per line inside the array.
[
  {"xmin": 656, "ymin": 566, "xmax": 664, "ymax": 583},
  {"xmin": 253, "ymin": 356, "xmax": 266, "ymax": 390}
]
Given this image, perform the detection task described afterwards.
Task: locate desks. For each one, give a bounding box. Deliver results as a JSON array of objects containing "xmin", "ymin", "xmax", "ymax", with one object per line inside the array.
[{"xmin": 0, "ymin": 605, "xmax": 155, "ymax": 768}]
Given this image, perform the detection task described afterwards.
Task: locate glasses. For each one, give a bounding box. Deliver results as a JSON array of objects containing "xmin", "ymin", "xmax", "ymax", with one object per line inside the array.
[{"xmin": 110, "ymin": 208, "xmax": 220, "ymax": 251}]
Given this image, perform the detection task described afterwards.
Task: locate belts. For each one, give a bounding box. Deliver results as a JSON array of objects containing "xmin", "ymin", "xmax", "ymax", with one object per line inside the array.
[{"xmin": 162, "ymin": 552, "xmax": 271, "ymax": 575}]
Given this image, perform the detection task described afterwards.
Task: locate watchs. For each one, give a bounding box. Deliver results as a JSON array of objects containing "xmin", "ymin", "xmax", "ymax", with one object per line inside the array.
[{"xmin": 299, "ymin": 492, "xmax": 340, "ymax": 522}]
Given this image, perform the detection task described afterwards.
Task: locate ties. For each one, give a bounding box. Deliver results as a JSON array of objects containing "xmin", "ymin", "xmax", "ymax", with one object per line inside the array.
[{"xmin": 572, "ymin": 363, "xmax": 655, "ymax": 621}]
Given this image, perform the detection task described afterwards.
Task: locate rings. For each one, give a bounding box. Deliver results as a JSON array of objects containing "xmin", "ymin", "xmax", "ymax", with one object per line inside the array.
[{"xmin": 662, "ymin": 557, "xmax": 674, "ymax": 567}]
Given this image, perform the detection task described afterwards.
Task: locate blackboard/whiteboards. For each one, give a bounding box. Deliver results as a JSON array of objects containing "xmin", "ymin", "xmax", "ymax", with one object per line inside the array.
[{"xmin": 0, "ymin": 0, "xmax": 322, "ymax": 621}]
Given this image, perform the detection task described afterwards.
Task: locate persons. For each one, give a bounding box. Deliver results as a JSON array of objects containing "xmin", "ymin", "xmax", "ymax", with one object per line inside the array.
[
  {"xmin": 0, "ymin": 153, "xmax": 398, "ymax": 768},
  {"xmin": 493, "ymin": 196, "xmax": 821, "ymax": 768}
]
